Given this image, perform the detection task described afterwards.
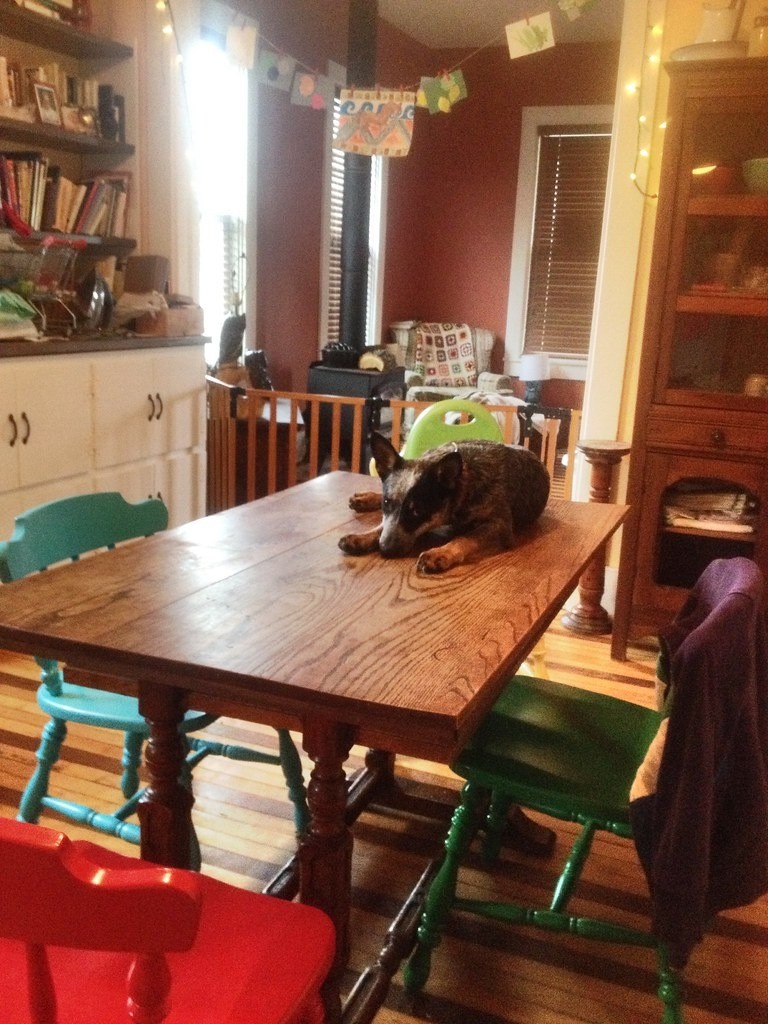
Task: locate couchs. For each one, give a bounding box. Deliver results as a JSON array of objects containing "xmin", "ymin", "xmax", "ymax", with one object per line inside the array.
[{"xmin": 388, "ymin": 320, "xmax": 515, "ymax": 432}]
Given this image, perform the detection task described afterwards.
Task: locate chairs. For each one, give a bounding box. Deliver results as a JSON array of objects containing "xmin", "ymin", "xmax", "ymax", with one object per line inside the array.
[
  {"xmin": 0, "ymin": 491, "xmax": 313, "ymax": 851},
  {"xmin": 0, "ymin": 816, "xmax": 336, "ymax": 1024},
  {"xmin": 402, "ymin": 557, "xmax": 768, "ymax": 1024},
  {"xmin": 400, "ymin": 399, "xmax": 506, "ymax": 460}
]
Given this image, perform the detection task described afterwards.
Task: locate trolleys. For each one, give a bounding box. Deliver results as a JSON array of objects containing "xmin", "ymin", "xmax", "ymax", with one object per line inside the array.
[{"xmin": 0, "ymin": 236, "xmax": 85, "ymax": 338}]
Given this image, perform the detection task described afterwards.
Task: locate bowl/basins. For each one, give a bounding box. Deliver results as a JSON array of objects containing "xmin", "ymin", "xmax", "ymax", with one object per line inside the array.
[{"xmin": 742, "ymin": 157, "xmax": 768, "ymax": 195}]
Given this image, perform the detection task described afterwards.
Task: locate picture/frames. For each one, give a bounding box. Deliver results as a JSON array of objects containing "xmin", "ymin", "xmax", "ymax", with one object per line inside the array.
[{"xmin": 30, "ymin": 80, "xmax": 65, "ymax": 130}]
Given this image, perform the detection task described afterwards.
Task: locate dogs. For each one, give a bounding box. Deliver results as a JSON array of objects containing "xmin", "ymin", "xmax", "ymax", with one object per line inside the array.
[{"xmin": 339, "ymin": 430, "xmax": 550, "ymax": 573}]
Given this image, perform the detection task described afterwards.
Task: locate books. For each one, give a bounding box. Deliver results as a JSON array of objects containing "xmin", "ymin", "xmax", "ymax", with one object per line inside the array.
[
  {"xmin": 0, "ymin": 56, "xmax": 126, "ymax": 238},
  {"xmin": 668, "ymin": 496, "xmax": 756, "ymax": 533}
]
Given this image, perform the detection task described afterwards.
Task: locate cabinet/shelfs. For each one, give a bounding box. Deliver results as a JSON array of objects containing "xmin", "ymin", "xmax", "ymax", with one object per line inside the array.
[
  {"xmin": 0, "ymin": 0, "xmax": 137, "ymax": 262},
  {"xmin": 0, "ymin": 352, "xmax": 94, "ymax": 578},
  {"xmin": 609, "ymin": 55, "xmax": 768, "ymax": 665},
  {"xmin": 93, "ymin": 343, "xmax": 208, "ymax": 555}
]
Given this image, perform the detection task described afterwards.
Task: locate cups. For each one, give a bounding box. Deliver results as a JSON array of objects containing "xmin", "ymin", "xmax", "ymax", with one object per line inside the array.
[{"xmin": 745, "ymin": 373, "xmax": 768, "ymax": 398}]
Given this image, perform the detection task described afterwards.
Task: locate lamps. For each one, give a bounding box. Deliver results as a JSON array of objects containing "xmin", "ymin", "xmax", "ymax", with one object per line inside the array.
[{"xmin": 517, "ymin": 353, "xmax": 551, "ymax": 404}]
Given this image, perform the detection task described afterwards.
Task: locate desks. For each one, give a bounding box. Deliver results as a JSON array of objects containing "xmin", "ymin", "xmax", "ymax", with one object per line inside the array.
[{"xmin": 0, "ymin": 468, "xmax": 632, "ymax": 1024}]
